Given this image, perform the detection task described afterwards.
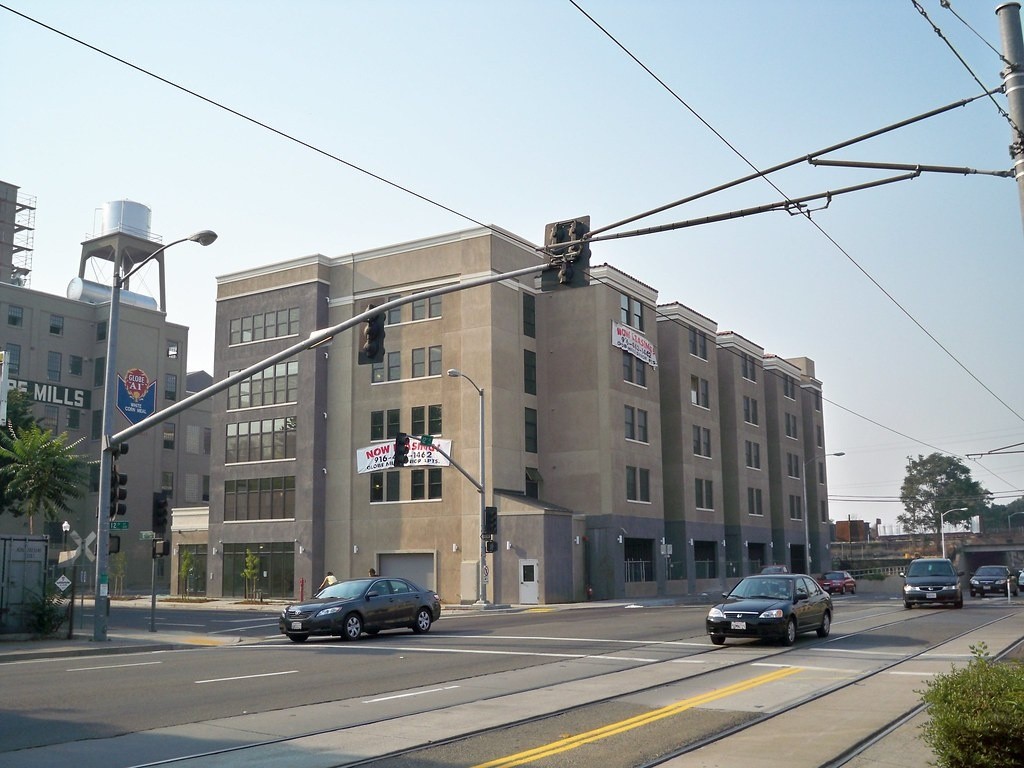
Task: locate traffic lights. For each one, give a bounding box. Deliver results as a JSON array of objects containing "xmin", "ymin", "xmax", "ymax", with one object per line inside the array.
[
  {"xmin": 153, "ymin": 492, "xmax": 168, "ymax": 533},
  {"xmin": 394, "ymin": 432, "xmax": 410, "ymax": 467},
  {"xmin": 110, "ymin": 471, "xmax": 127, "ymax": 521},
  {"xmin": 486, "ymin": 507, "xmax": 497, "ymax": 535},
  {"xmin": 114, "ymin": 443, "xmax": 129, "ymax": 461},
  {"xmin": 110, "ymin": 536, "xmax": 120, "ymax": 553},
  {"xmin": 487, "ymin": 541, "xmax": 498, "ymax": 551}
]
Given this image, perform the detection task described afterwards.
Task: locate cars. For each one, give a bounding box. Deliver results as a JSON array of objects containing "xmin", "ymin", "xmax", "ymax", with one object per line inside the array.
[
  {"xmin": 279, "ymin": 577, "xmax": 442, "ymax": 643},
  {"xmin": 706, "ymin": 565, "xmax": 834, "ymax": 645},
  {"xmin": 969, "ymin": 565, "xmax": 1019, "ymax": 598},
  {"xmin": 816, "ymin": 571, "xmax": 858, "ymax": 595},
  {"xmin": 1018, "ymin": 567, "xmax": 1024, "ymax": 593}
]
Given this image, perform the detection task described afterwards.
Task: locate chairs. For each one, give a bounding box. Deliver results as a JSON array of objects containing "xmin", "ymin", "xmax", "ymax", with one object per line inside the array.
[
  {"xmin": 931, "ymin": 565, "xmax": 946, "ymax": 573},
  {"xmin": 756, "ymin": 583, "xmax": 780, "ymax": 598},
  {"xmin": 982, "ymin": 570, "xmax": 993, "ymax": 575},
  {"xmin": 995, "ymin": 572, "xmax": 1002, "ymax": 576},
  {"xmin": 914, "ymin": 564, "xmax": 928, "ymax": 574}
]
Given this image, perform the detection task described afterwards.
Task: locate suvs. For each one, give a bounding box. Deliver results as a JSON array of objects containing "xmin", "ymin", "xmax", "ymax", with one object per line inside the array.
[{"xmin": 900, "ymin": 556, "xmax": 965, "ymax": 609}]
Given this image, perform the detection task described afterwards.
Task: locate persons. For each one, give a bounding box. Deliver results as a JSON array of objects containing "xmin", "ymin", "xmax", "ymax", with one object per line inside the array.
[
  {"xmin": 369, "ymin": 568, "xmax": 375, "ymax": 577},
  {"xmin": 320, "ymin": 572, "xmax": 340, "ymax": 589}
]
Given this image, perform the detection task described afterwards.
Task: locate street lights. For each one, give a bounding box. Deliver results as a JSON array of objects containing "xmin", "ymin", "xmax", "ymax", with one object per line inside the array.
[
  {"xmin": 1008, "ymin": 512, "xmax": 1024, "ymax": 568},
  {"xmin": 447, "ymin": 369, "xmax": 486, "ymax": 600},
  {"xmin": 803, "ymin": 452, "xmax": 845, "ymax": 577},
  {"xmin": 61, "ymin": 520, "xmax": 70, "ymax": 599},
  {"xmin": 940, "ymin": 507, "xmax": 968, "ymax": 560},
  {"xmin": 95, "ymin": 230, "xmax": 218, "ymax": 639}
]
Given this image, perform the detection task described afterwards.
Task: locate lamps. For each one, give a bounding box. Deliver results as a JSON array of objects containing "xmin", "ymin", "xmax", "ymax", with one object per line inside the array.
[
  {"xmin": 212, "ymin": 547, "xmax": 217, "ymax": 556},
  {"xmin": 354, "ymin": 545, "xmax": 359, "ymax": 554},
  {"xmin": 574, "ymin": 535, "xmax": 580, "ymax": 545},
  {"xmin": 660, "ymin": 537, "xmax": 665, "ymax": 545},
  {"xmin": 323, "ymin": 412, "xmax": 327, "ymax": 420},
  {"xmin": 744, "ymin": 540, "xmax": 749, "ymax": 547},
  {"xmin": 787, "ymin": 542, "xmax": 790, "ymax": 549},
  {"xmin": 808, "ymin": 543, "xmax": 812, "ymax": 549},
  {"xmin": 299, "ymin": 545, "xmax": 305, "ymax": 554},
  {"xmin": 173, "ymin": 549, "xmax": 179, "ymax": 556},
  {"xmin": 721, "ymin": 540, "xmax": 726, "ymax": 546},
  {"xmin": 322, "ymin": 468, "xmax": 327, "ymax": 475},
  {"xmin": 825, "ymin": 544, "xmax": 829, "ymax": 550},
  {"xmin": 688, "ymin": 539, "xmax": 693, "ymax": 546},
  {"xmin": 770, "ymin": 542, "xmax": 773, "ymax": 548},
  {"xmin": 507, "ymin": 541, "xmax": 513, "ymax": 551},
  {"xmin": 326, "ymin": 297, "xmax": 330, "ymax": 302},
  {"xmin": 453, "ymin": 543, "xmax": 458, "ymax": 553},
  {"xmin": 324, "ymin": 352, "xmax": 329, "ymax": 360},
  {"xmin": 617, "ymin": 535, "xmax": 623, "ymax": 544}
]
